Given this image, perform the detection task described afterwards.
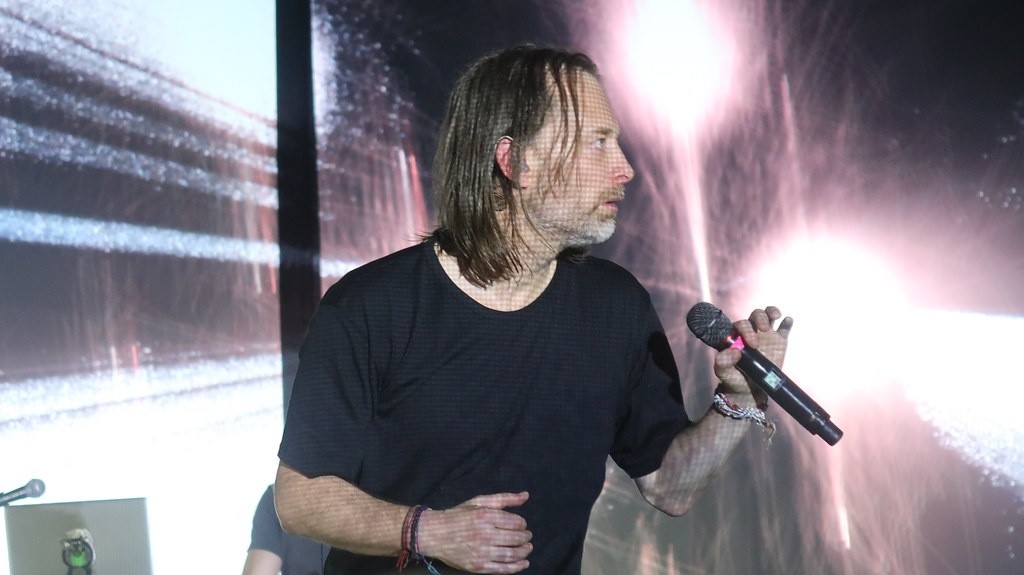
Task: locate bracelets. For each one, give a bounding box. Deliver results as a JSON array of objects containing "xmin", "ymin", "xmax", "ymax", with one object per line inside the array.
[
  {"xmin": 394, "ymin": 505, "xmax": 442, "ymax": 575},
  {"xmin": 712, "ymin": 383, "xmax": 778, "ymax": 445}
]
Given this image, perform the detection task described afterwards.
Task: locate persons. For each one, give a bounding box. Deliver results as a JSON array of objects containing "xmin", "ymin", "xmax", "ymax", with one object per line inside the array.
[
  {"xmin": 242, "ymin": 484, "xmax": 331, "ymax": 575},
  {"xmin": 273, "ymin": 44, "xmax": 793, "ymax": 575}
]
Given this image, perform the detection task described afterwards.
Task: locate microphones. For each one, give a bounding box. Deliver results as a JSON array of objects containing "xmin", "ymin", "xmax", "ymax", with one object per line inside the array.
[
  {"xmin": 686, "ymin": 302, "xmax": 844, "ymax": 446},
  {"xmin": 0, "ymin": 478, "xmax": 45, "ymax": 506}
]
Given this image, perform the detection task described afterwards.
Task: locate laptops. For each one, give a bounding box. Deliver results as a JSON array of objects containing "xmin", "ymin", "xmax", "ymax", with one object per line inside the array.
[{"xmin": 6, "ymin": 497, "xmax": 152, "ymax": 575}]
